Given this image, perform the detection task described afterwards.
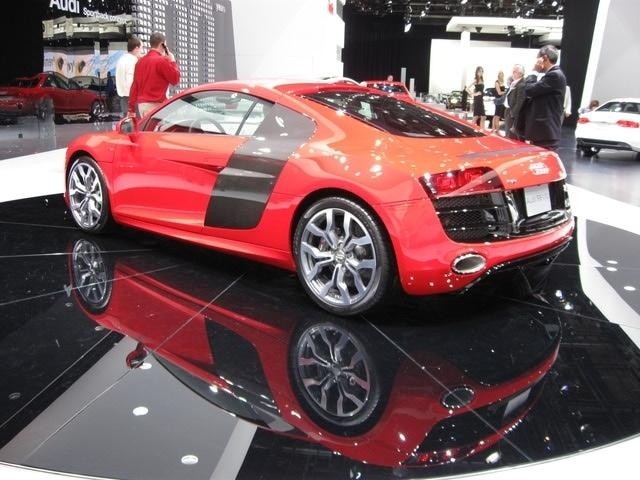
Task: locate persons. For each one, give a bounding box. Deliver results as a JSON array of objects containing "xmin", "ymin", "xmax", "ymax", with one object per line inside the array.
[
  {"xmin": 127, "ymin": 33, "xmax": 180, "ymax": 119},
  {"xmin": 461, "ymin": 44, "xmax": 572, "ymax": 153},
  {"xmin": 386, "ymin": 75, "xmax": 394, "ymax": 82},
  {"xmin": 105, "ymin": 72, "xmax": 114, "ymax": 112},
  {"xmin": 116, "ymin": 38, "xmax": 143, "ymax": 117},
  {"xmin": 578, "ymin": 100, "xmax": 599, "ymax": 116}
]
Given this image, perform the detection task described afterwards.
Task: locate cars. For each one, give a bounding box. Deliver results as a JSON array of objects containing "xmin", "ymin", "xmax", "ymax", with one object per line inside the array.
[
  {"xmin": 443, "ymin": 83, "xmax": 526, "ymax": 122},
  {"xmin": 361, "ymin": 76, "xmax": 418, "ymax": 106},
  {"xmin": 318, "ymin": 73, "xmax": 361, "ymax": 87},
  {"xmin": 575, "ymin": 94, "xmax": 640, "ymax": 166},
  {"xmin": 1, "ymin": 69, "xmax": 107, "ymax": 121}
]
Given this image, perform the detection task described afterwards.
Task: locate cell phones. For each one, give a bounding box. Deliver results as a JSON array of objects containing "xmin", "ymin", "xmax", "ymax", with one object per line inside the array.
[{"xmin": 162, "ymin": 43, "xmax": 168, "ymax": 55}]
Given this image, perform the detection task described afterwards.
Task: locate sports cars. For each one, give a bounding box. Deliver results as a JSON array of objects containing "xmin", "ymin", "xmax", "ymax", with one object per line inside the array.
[
  {"xmin": 61, "ymin": 76, "xmax": 579, "ymax": 319},
  {"xmin": 66, "ymin": 238, "xmax": 565, "ymax": 466}
]
